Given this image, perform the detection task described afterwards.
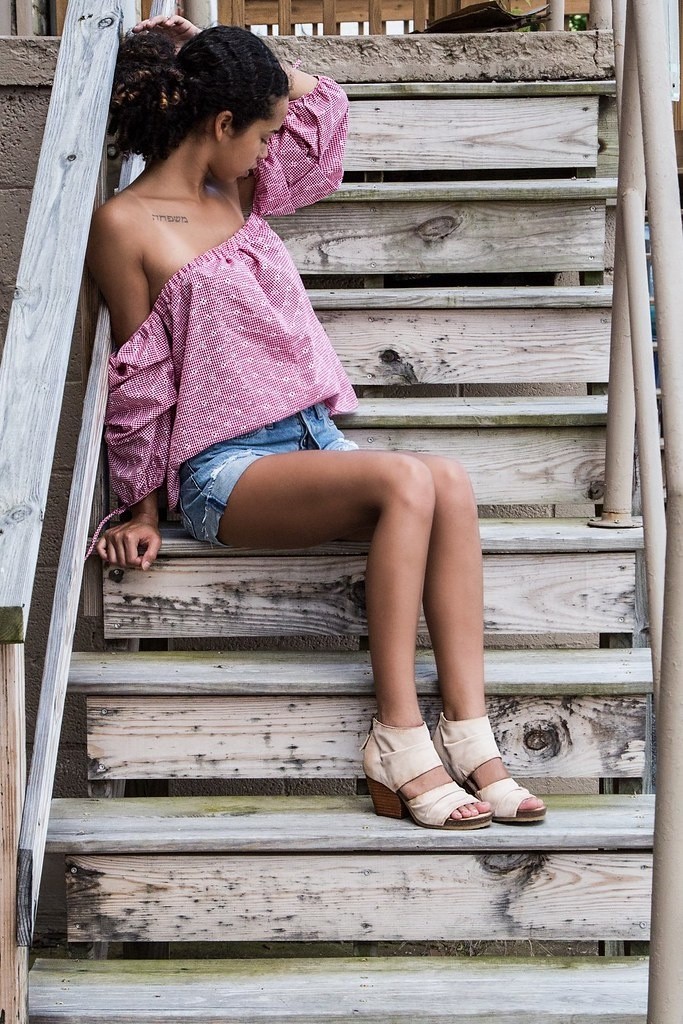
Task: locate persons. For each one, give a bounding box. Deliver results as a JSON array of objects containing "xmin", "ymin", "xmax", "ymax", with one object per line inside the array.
[{"xmin": 86, "ymin": 15, "xmax": 547, "ymax": 831}]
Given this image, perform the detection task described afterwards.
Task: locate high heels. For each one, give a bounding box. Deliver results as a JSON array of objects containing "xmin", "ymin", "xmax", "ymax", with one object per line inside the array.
[
  {"xmin": 358, "ymin": 714, "xmax": 492, "ymax": 830},
  {"xmin": 432, "ymin": 712, "xmax": 546, "ymax": 823}
]
